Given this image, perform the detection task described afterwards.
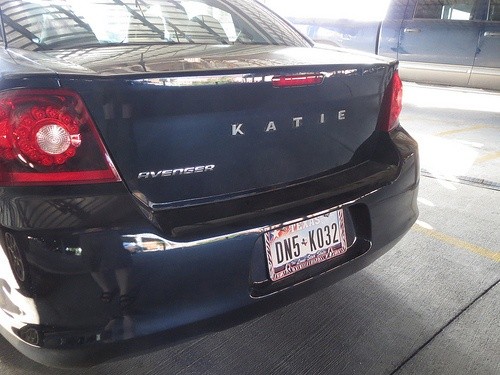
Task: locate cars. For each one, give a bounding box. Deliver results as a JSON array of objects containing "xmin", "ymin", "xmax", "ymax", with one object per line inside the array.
[{"xmin": 2, "ymin": 0, "xmax": 419, "ymax": 370}]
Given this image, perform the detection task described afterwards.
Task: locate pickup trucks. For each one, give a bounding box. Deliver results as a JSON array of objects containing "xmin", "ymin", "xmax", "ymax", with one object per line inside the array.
[{"xmin": 285, "ymin": 0, "xmax": 499, "ymax": 93}]
